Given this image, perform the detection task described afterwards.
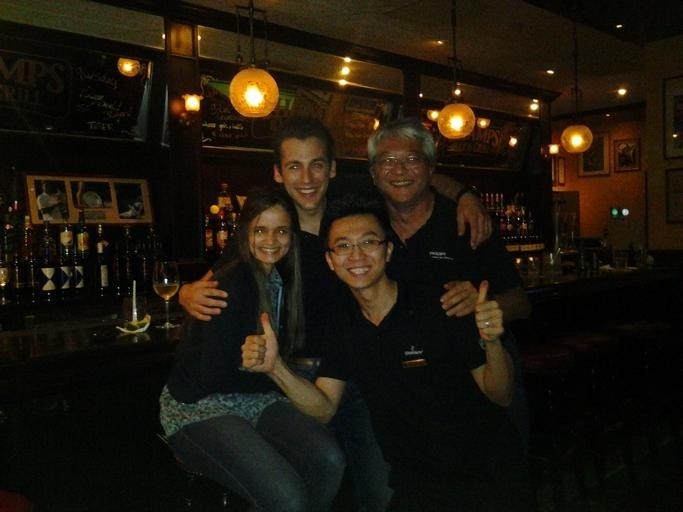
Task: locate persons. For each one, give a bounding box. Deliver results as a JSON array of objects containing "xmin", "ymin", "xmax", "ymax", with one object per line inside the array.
[
  {"xmin": 242, "ymin": 191, "xmax": 532, "ymax": 512},
  {"xmin": 177, "ymin": 116, "xmax": 390, "ymax": 512},
  {"xmin": 55, "ymin": 188, "xmax": 68, "ymax": 219},
  {"xmin": 365, "ymin": 117, "xmax": 534, "ymax": 321},
  {"xmin": 36, "ymin": 180, "xmax": 63, "ymax": 220},
  {"xmin": 150, "ymin": 188, "xmax": 348, "ymax": 511}
]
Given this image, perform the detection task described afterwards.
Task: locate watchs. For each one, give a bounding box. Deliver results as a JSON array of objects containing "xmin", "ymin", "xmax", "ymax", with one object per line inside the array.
[{"xmin": 457, "ymin": 188, "xmax": 481, "ymax": 202}]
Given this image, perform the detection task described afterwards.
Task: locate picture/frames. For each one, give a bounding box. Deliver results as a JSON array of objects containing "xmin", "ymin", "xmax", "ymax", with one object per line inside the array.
[
  {"xmin": 613, "ymin": 137, "xmax": 641, "ymax": 173},
  {"xmin": 552, "ymin": 156, "xmax": 556, "ymax": 186},
  {"xmin": 578, "ymin": 128, "xmax": 610, "ymax": 177},
  {"xmin": 558, "ymin": 157, "xmax": 566, "ymax": 186},
  {"xmin": 662, "ymin": 75, "xmax": 683, "ymax": 160},
  {"xmin": 26, "ymin": 175, "xmax": 152, "ymax": 224},
  {"xmin": 665, "ymin": 168, "xmax": 683, "ymax": 224}
]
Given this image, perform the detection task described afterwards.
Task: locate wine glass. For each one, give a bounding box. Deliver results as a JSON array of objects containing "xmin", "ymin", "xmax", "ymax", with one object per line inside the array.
[
  {"xmin": 152, "ymin": 262, "xmax": 179, "ymax": 330},
  {"xmin": 529, "ymin": 254, "xmax": 542, "ymax": 287},
  {"xmin": 544, "ymin": 252, "xmax": 560, "ymax": 284}
]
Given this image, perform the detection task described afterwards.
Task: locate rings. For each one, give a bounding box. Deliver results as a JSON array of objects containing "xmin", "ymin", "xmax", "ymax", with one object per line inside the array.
[{"xmin": 485, "ymin": 320, "xmax": 490, "ymax": 328}]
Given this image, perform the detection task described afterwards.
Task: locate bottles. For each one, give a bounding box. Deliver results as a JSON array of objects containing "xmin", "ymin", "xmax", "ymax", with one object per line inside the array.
[
  {"xmin": 118, "ymin": 225, "xmax": 138, "ymax": 294},
  {"xmin": 95, "ymin": 224, "xmax": 113, "ymax": 295},
  {"xmin": 138, "ymin": 226, "xmax": 152, "ymax": 295},
  {"xmin": 479, "ymin": 191, "xmax": 528, "ymax": 239},
  {"xmin": 76, "ymin": 225, "xmax": 94, "ymax": 297},
  {"xmin": 60, "ymin": 225, "xmax": 75, "ymax": 301},
  {"xmin": 218, "ymin": 183, "xmax": 235, "ymax": 213},
  {"xmin": 215, "ymin": 211, "xmax": 231, "ymax": 258},
  {"xmin": 38, "ymin": 221, "xmax": 55, "ymax": 303},
  {"xmin": 21, "ymin": 214, "xmax": 37, "ymax": 303},
  {"xmin": 231, "ymin": 212, "xmax": 239, "ymax": 258},
  {"xmin": 205, "ymin": 213, "xmax": 213, "ymax": 259}
]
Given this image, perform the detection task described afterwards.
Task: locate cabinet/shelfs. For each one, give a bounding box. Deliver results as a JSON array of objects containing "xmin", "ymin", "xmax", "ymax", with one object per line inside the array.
[{"xmin": 0, "ymin": 0, "xmax": 562, "ymax": 288}]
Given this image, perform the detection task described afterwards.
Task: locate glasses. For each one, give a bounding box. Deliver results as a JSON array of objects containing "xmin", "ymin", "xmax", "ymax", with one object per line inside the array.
[
  {"xmin": 330, "ymin": 239, "xmax": 389, "ymax": 257},
  {"xmin": 369, "ymin": 156, "xmax": 422, "ymax": 167}
]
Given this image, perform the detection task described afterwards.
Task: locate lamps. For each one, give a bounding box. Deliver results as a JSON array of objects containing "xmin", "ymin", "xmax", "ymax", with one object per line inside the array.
[
  {"xmin": 181, "ymin": 70, "xmax": 217, "ymax": 112},
  {"xmin": 228, "ymin": 0, "xmax": 280, "ymax": 118},
  {"xmin": 436, "ymin": 0, "xmax": 476, "ymax": 140},
  {"xmin": 560, "ymin": 1, "xmax": 593, "ymax": 154}
]
